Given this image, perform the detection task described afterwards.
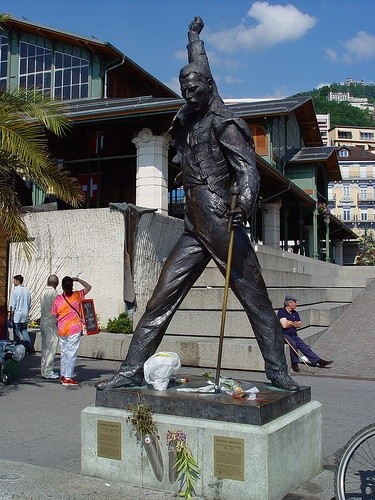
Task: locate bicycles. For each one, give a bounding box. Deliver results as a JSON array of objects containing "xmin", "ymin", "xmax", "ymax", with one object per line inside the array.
[{"xmin": 334, "ymin": 423, "xmax": 375, "ymax": 500}]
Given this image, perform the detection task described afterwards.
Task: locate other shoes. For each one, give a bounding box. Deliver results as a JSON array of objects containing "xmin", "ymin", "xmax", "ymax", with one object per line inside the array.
[
  {"xmin": 41, "ymin": 372, "xmax": 60, "ymax": 379},
  {"xmin": 292, "ymin": 365, "xmax": 300, "ymax": 372},
  {"xmin": 319, "ymin": 360, "xmax": 333, "ymax": 368},
  {"xmin": 61, "ymin": 376, "xmax": 79, "ymax": 385}
]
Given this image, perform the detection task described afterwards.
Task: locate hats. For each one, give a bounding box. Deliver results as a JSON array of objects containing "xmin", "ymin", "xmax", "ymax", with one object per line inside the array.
[{"xmin": 285, "ymin": 295, "xmax": 298, "ymax": 301}]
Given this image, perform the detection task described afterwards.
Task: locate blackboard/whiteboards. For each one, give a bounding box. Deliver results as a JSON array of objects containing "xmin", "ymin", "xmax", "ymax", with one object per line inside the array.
[{"xmin": 82, "ymin": 299, "xmax": 99, "ymax": 335}]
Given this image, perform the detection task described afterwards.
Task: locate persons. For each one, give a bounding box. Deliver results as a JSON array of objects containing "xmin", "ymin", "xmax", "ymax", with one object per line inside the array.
[
  {"xmin": 52, "ymin": 276, "xmax": 92, "ymax": 385},
  {"xmin": 95, "ymin": 15, "xmax": 297, "ymax": 392},
  {"xmin": 277, "ymin": 296, "xmax": 335, "ymax": 372},
  {"xmin": 9, "ymin": 274, "xmax": 36, "ymax": 355},
  {"xmin": 39, "ymin": 275, "xmax": 60, "ymax": 379}
]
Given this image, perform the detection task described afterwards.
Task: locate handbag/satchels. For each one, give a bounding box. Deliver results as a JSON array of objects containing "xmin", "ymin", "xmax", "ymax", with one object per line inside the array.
[{"xmin": 82, "ymin": 324, "xmax": 87, "ymax": 335}]
